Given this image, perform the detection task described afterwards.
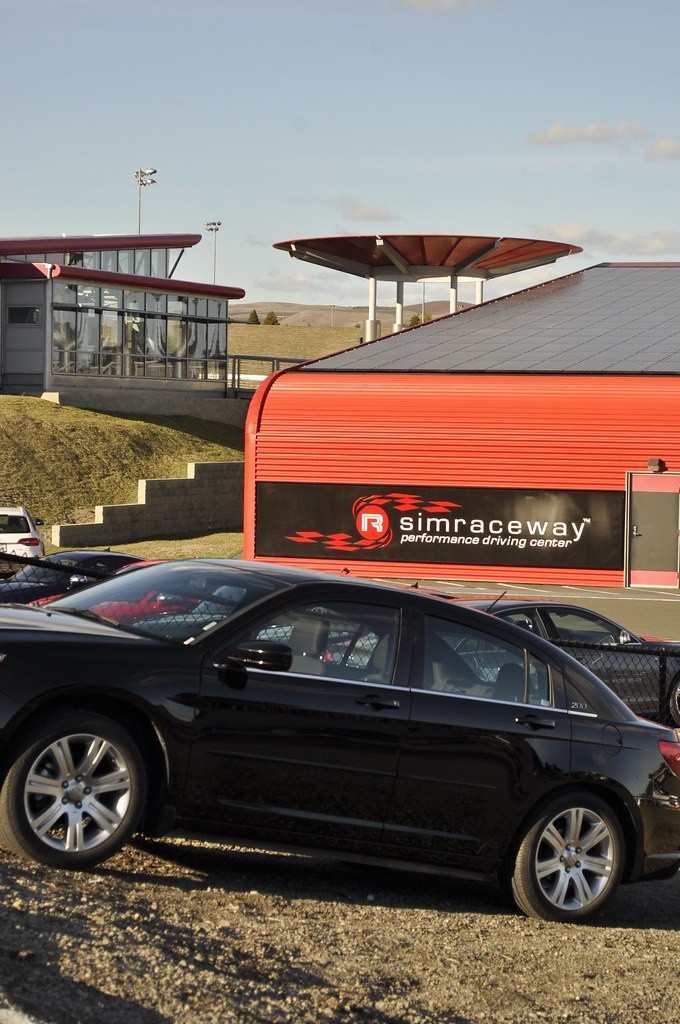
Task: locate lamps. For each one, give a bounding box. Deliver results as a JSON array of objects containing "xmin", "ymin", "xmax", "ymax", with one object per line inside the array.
[{"xmin": 648, "ymin": 458, "xmax": 663, "ymax": 472}]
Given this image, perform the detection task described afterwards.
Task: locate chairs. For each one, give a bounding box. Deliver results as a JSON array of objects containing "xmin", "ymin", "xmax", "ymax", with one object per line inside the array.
[
  {"xmin": 288, "ymin": 617, "xmax": 328, "ymax": 677},
  {"xmin": 363, "ymin": 635, "xmax": 392, "ymax": 682},
  {"xmin": 5, "ymin": 517, "xmax": 23, "ymax": 531},
  {"xmin": 428, "ymin": 661, "xmax": 537, "ymax": 705}
]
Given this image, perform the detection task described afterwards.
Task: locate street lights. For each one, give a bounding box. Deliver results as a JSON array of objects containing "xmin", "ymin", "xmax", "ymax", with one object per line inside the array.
[
  {"xmin": 135, "ymin": 167, "xmax": 159, "ymax": 237},
  {"xmin": 202, "ymin": 219, "xmax": 224, "ymax": 288}
]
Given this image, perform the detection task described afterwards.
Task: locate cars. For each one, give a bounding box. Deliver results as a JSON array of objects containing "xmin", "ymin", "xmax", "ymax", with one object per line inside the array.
[
  {"xmin": 0, "ymin": 559, "xmax": 680, "ymax": 921},
  {"xmin": 0, "ymin": 504, "xmax": 48, "ymax": 564},
  {"xmin": 0, "ymin": 547, "xmax": 362, "ymax": 642},
  {"xmin": 327, "ymin": 598, "xmax": 679, "ymax": 733}
]
